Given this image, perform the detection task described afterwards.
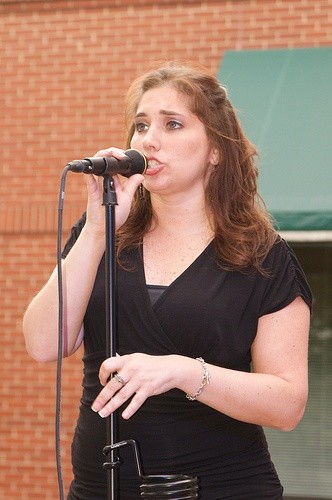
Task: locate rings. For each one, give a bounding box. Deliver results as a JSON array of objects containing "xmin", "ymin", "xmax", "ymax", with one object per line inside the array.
[{"xmin": 114, "ymin": 373, "xmax": 127, "ymax": 386}]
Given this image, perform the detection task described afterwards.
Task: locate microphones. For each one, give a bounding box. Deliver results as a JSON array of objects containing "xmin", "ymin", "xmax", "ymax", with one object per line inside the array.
[{"xmin": 67, "ymin": 149, "xmax": 148, "ymax": 179}]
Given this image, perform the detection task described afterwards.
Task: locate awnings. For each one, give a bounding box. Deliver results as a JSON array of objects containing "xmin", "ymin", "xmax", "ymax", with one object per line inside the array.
[{"xmin": 214, "ymin": 46, "xmax": 332, "ymax": 245}]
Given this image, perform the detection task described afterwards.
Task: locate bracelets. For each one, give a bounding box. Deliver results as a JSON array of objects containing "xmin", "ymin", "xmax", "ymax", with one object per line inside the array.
[{"xmin": 186, "ymin": 357, "xmax": 210, "ymax": 401}]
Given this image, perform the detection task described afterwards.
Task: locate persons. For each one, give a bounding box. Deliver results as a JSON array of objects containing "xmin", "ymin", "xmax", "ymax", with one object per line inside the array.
[{"xmin": 20, "ymin": 66, "xmax": 314, "ymax": 500}]
